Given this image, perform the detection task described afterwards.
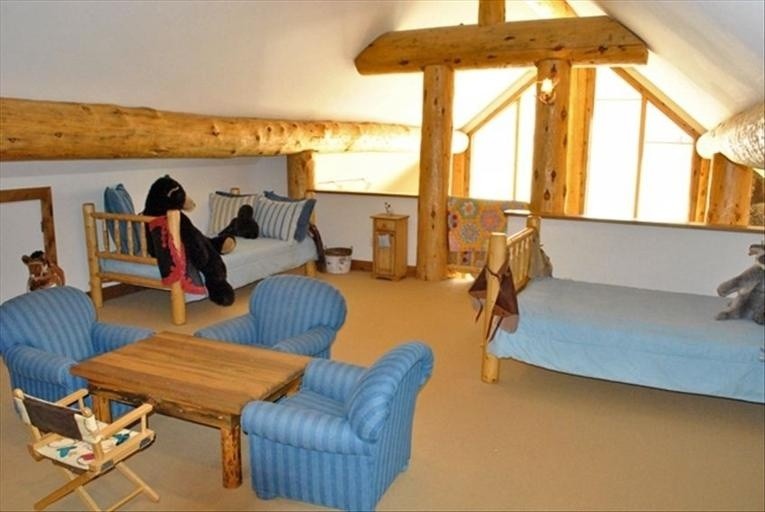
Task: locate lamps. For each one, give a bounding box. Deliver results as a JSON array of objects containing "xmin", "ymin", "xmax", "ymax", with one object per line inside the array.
[{"xmin": 539, "ymin": 77, "xmax": 557, "ymax": 106}]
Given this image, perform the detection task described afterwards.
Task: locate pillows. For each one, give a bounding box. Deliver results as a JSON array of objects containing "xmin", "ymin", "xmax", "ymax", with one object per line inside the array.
[
  {"xmin": 208, "ymin": 192, "xmax": 256, "ymax": 234},
  {"xmin": 216, "ymin": 190, "xmax": 256, "ymax": 198},
  {"xmin": 263, "ymin": 190, "xmax": 317, "ymax": 243},
  {"xmin": 253, "ymin": 194, "xmax": 307, "ymax": 243}
]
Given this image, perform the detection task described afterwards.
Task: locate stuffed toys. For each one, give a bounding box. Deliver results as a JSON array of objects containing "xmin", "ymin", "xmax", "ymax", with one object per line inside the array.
[
  {"xmin": 712, "ymin": 242, "xmax": 761, "ymax": 325},
  {"xmin": 136, "ymin": 174, "xmax": 260, "ymax": 307}
]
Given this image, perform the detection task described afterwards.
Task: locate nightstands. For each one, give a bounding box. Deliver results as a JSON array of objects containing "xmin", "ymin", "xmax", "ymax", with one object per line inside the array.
[{"xmin": 370, "ymin": 213, "xmax": 410, "ymax": 281}]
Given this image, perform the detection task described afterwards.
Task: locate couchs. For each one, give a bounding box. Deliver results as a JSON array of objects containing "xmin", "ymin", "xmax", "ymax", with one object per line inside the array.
[
  {"xmin": 194, "ymin": 275, "xmax": 346, "ymax": 361},
  {"xmin": 0, "ymin": 287, "xmax": 156, "ymax": 425},
  {"xmin": 239, "ymin": 342, "xmax": 434, "ymax": 512}
]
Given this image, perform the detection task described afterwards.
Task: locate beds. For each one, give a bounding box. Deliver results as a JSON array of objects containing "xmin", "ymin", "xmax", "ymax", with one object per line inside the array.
[
  {"xmin": 467, "ymin": 214, "xmax": 765, "ymax": 404},
  {"xmin": 82, "ymin": 187, "xmax": 317, "ymax": 326}
]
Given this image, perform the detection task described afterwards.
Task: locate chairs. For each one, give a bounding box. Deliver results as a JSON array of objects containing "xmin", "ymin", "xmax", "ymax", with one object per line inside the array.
[{"xmin": 12, "ymin": 388, "xmax": 159, "ymax": 512}]
